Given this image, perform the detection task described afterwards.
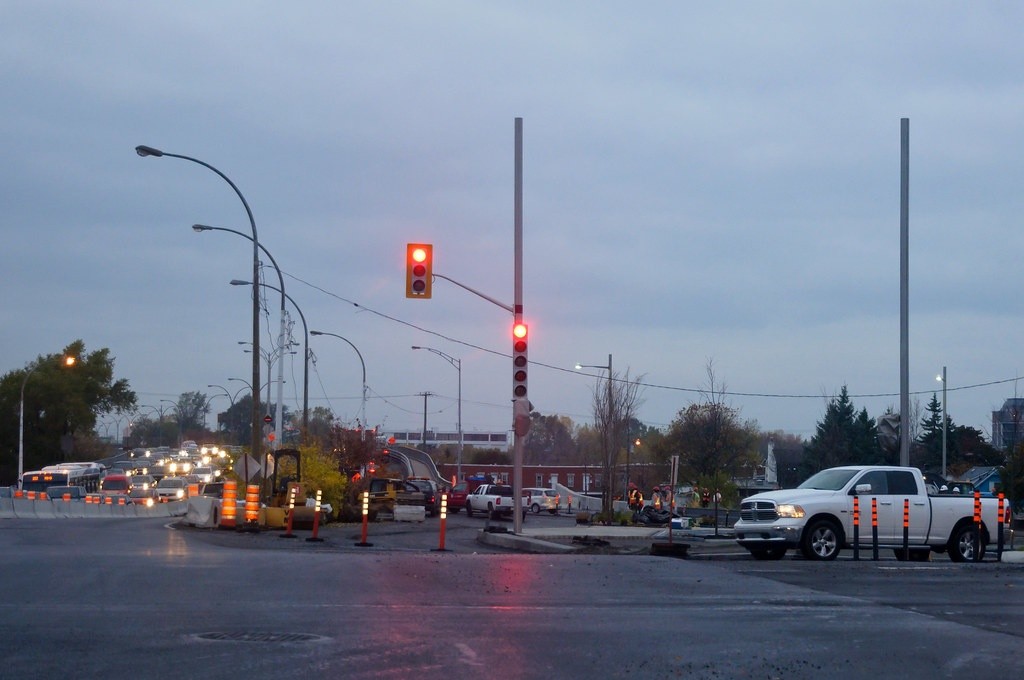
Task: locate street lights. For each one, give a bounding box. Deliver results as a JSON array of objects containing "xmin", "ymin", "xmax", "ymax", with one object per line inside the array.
[
  {"xmin": 936, "ymin": 366, "xmax": 948, "ymax": 480},
  {"xmin": 575, "ymin": 355, "xmax": 616, "ymax": 522},
  {"xmin": 310, "ymin": 330, "xmax": 366, "ymax": 425},
  {"xmin": 94, "ymin": 338, "xmax": 301, "ymax": 452},
  {"xmin": 132, "ymin": 144, "xmax": 263, "ymax": 483},
  {"xmin": 191, "ymin": 222, "xmax": 284, "ymax": 445},
  {"xmin": 410, "ymin": 345, "xmax": 462, "ymax": 479},
  {"xmin": 228, "ymin": 278, "xmax": 310, "ymax": 444},
  {"xmin": 17, "ymin": 357, "xmax": 74, "ymax": 489}
]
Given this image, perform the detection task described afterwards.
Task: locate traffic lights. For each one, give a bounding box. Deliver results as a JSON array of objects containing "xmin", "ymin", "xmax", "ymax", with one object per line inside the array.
[
  {"xmin": 511, "ymin": 322, "xmax": 529, "ymax": 403},
  {"xmin": 405, "ymin": 243, "xmax": 434, "ymax": 300}
]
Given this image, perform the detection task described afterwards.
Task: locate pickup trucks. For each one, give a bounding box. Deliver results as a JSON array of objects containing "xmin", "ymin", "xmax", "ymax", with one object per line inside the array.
[{"xmin": 735, "ymin": 464, "xmax": 1011, "ymax": 565}]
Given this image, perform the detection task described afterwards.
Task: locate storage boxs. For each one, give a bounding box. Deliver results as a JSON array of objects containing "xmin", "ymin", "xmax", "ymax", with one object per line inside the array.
[{"xmin": 671, "ymin": 517, "xmax": 693, "ymax": 530}]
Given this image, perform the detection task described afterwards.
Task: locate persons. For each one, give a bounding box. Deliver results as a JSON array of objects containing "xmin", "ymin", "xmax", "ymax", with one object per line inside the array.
[
  {"xmin": 692, "ymin": 487, "xmax": 700, "ymax": 502},
  {"xmin": 992, "ymin": 488, "xmax": 996, "ymax": 496},
  {"xmin": 952, "ymin": 487, "xmax": 960, "ymax": 494},
  {"xmin": 702, "ymin": 488, "xmax": 710, "ymax": 508},
  {"xmin": 651, "ymin": 487, "xmax": 663, "ymax": 509},
  {"xmin": 664, "ymin": 486, "xmax": 675, "ymax": 509},
  {"xmin": 627, "ymin": 483, "xmax": 643, "ymax": 524},
  {"xmin": 940, "ymin": 485, "xmax": 948, "ymax": 494},
  {"xmin": 713, "ymin": 489, "xmax": 721, "ymax": 508}
]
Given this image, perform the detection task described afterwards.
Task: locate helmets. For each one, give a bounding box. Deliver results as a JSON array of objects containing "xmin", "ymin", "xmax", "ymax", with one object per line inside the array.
[
  {"xmin": 632, "ymin": 485, "xmax": 637, "ymax": 490},
  {"xmin": 665, "ymin": 485, "xmax": 670, "ymax": 490},
  {"xmin": 693, "ymin": 487, "xmax": 698, "ymax": 491},
  {"xmin": 653, "ymin": 486, "xmax": 659, "ymax": 490},
  {"xmin": 628, "ymin": 483, "xmax": 634, "ymax": 489}
]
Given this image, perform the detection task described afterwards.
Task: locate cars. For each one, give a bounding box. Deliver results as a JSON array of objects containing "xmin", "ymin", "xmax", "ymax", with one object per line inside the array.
[
  {"xmin": 372, "ymin": 478, "xmax": 561, "ymax": 522},
  {"xmin": 22, "ymin": 445, "xmax": 233, "ymax": 508}
]
